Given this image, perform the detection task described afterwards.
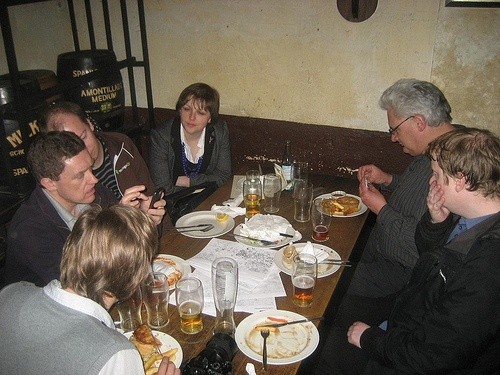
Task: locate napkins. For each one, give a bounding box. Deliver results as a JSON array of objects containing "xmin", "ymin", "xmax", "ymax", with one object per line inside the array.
[
  {"xmin": 297, "ymin": 241, "xmax": 329, "ymax": 264},
  {"xmin": 211, "ymin": 204, "xmax": 246, "ymax": 218}
]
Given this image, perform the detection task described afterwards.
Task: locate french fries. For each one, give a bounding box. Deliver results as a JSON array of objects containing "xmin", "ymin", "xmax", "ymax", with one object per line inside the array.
[
  {"xmin": 254, "ymin": 326, "xmax": 275, "ymax": 332},
  {"xmin": 143, "ymin": 349, "xmax": 178, "ymax": 375}
]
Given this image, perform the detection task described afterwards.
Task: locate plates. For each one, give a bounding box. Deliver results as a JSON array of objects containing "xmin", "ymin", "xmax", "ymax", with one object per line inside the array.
[
  {"xmin": 273, "ymin": 243, "xmax": 342, "ymax": 279},
  {"xmin": 149, "ymin": 252, "xmax": 192, "ymax": 294},
  {"xmin": 234, "ymin": 309, "xmax": 320, "ymax": 365},
  {"xmin": 233, "ymin": 223, "xmax": 291, "ymax": 249},
  {"xmin": 314, "ymin": 193, "xmax": 368, "ymax": 218},
  {"xmin": 175, "ymin": 210, "xmax": 235, "ymax": 239},
  {"xmin": 122, "ymin": 331, "xmax": 183, "ymax": 369}
]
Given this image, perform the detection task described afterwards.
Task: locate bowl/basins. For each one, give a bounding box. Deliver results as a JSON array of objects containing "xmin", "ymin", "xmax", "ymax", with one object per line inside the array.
[{"xmin": 331, "ymin": 190, "xmax": 346, "ymax": 198}]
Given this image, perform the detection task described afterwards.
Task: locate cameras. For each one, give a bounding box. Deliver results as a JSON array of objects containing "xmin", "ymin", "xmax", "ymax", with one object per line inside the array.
[{"xmin": 179, "ymin": 333, "xmax": 238, "ymax": 375}]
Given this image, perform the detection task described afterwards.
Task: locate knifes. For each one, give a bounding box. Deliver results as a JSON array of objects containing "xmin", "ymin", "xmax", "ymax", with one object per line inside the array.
[
  {"xmin": 259, "ymin": 317, "xmax": 324, "ymax": 328},
  {"xmin": 177, "ymin": 224, "xmax": 211, "ymax": 229}
]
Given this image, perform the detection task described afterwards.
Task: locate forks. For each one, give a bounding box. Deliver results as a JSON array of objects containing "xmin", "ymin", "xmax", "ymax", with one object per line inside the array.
[
  {"xmin": 179, "ymin": 225, "xmax": 214, "ymax": 233},
  {"xmin": 261, "ymin": 329, "xmax": 270, "ymax": 370}
]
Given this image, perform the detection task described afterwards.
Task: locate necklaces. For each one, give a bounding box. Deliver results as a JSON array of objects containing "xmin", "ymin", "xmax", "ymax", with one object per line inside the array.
[{"xmin": 182, "ymin": 142, "xmax": 203, "ymax": 179}]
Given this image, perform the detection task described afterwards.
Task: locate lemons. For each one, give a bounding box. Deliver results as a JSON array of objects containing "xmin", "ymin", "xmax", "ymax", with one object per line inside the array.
[{"xmin": 215, "ymin": 213, "xmax": 228, "ymax": 221}]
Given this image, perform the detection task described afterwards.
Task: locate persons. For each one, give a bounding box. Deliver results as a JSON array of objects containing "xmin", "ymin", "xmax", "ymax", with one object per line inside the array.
[
  {"xmin": 294, "ymin": 127, "xmax": 500, "ymax": 375},
  {"xmin": 39, "ymin": 100, "xmax": 173, "ymax": 229},
  {"xmin": 0, "ymin": 204, "xmax": 180, "ymax": 375},
  {"xmin": 6, "ymin": 131, "xmax": 166, "ymax": 288},
  {"xmin": 147, "ymin": 83, "xmax": 233, "ymax": 227},
  {"xmin": 318, "ymin": 78, "xmax": 467, "ymax": 327}
]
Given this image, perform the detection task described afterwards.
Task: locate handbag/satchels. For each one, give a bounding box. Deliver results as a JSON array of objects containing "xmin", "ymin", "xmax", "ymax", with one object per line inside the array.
[{"xmin": 162, "ymin": 180, "xmax": 219, "ymax": 227}]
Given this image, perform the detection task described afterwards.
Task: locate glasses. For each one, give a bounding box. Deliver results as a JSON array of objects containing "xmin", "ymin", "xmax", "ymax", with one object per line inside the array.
[{"xmin": 389, "ymin": 116, "xmax": 417, "ymax": 137}]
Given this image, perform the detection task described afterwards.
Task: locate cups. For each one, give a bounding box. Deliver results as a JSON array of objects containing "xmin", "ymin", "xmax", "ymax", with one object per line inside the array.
[
  {"xmin": 290, "ymin": 253, "xmax": 318, "ymax": 307},
  {"xmin": 211, "ymin": 258, "xmax": 238, "ymax": 340},
  {"xmin": 291, "ymin": 161, "xmax": 308, "ymax": 198},
  {"xmin": 311, "ymin": 204, "xmax": 333, "ymax": 243},
  {"xmin": 174, "ymin": 278, "xmax": 204, "ymax": 335},
  {"xmin": 242, "ymin": 179, "xmax": 262, "ymax": 219},
  {"xmin": 141, "ymin": 272, "xmax": 170, "ymax": 330},
  {"xmin": 263, "ymin": 173, "xmax": 282, "ymax": 213},
  {"xmin": 292, "ymin": 180, "xmax": 313, "ymax": 222},
  {"xmin": 114, "ymin": 286, "xmax": 142, "ymax": 332},
  {"xmin": 246, "ymin": 169, "xmax": 259, "ymax": 180}
]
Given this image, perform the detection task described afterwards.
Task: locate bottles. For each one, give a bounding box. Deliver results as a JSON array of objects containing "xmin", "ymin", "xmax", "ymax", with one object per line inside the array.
[{"xmin": 281, "ymin": 140, "xmax": 295, "ymax": 192}]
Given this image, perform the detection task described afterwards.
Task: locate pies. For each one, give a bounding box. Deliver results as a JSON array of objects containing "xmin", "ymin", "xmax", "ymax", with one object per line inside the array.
[
  {"xmin": 334, "ymin": 196, "xmax": 361, "ymax": 212},
  {"xmin": 129, "ymin": 323, "xmax": 162, "ymax": 356}
]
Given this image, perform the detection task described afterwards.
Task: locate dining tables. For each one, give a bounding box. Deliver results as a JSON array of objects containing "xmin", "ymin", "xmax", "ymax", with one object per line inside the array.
[{"xmin": 110, "ymin": 162, "xmax": 385, "ymax": 375}]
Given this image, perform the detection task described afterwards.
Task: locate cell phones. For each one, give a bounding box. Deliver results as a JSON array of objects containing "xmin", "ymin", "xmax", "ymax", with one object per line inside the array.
[{"xmin": 149, "ymin": 187, "xmax": 165, "ymax": 209}]
